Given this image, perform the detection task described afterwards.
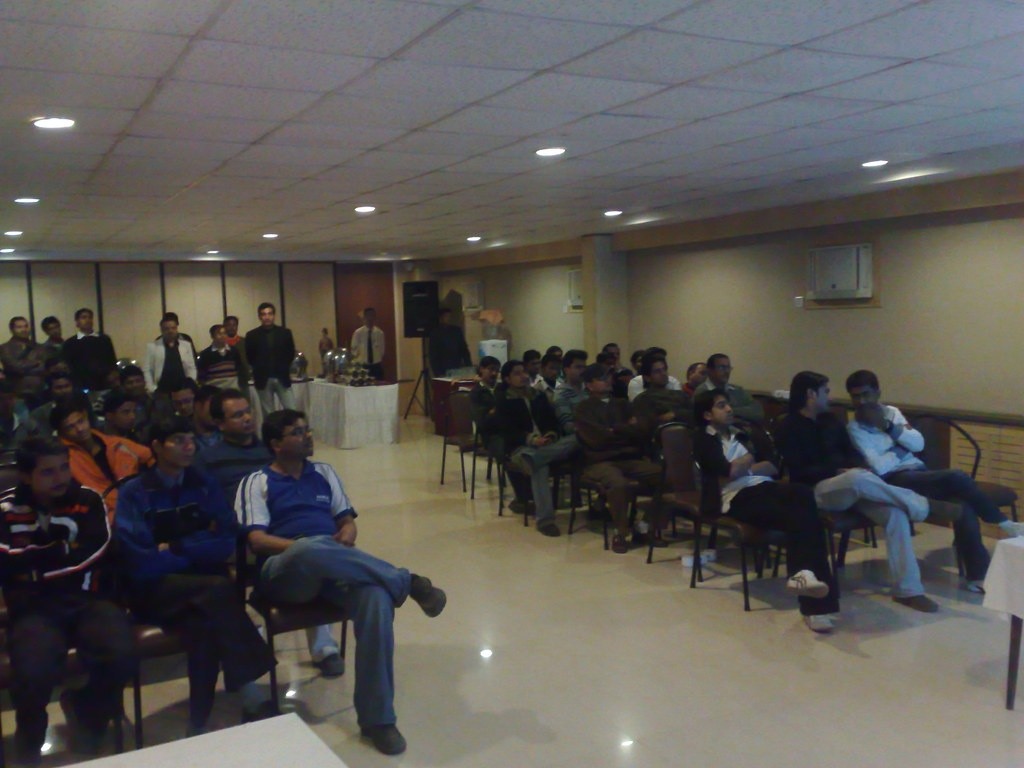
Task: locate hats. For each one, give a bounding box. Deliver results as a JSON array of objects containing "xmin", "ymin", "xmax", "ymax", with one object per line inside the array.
[{"xmin": 580, "ymin": 362, "xmax": 615, "ymax": 382}]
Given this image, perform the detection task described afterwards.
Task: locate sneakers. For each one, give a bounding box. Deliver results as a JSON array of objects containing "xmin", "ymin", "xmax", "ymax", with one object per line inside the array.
[
  {"xmin": 983, "ymin": 520, "xmax": 1024, "ymax": 538},
  {"xmin": 361, "ymin": 725, "xmax": 406, "ymax": 755},
  {"xmin": 895, "ymin": 595, "xmax": 939, "ymax": 612},
  {"xmin": 925, "ymin": 498, "xmax": 962, "ymax": 525},
  {"xmin": 410, "ymin": 575, "xmax": 446, "ymax": 618},
  {"xmin": 804, "ymin": 614, "xmax": 832, "ymax": 631},
  {"xmin": 967, "ymin": 579, "xmax": 984, "ymax": 592},
  {"xmin": 785, "ymin": 569, "xmax": 829, "ymax": 599}
]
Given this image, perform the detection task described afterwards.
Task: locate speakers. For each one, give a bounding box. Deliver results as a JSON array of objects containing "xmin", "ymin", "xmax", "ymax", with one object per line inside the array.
[{"xmin": 403, "ymin": 280, "xmax": 441, "ymax": 337}]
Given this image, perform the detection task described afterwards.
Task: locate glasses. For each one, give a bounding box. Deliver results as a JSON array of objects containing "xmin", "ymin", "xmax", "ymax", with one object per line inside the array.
[{"xmin": 275, "ymin": 426, "xmax": 311, "ymax": 441}]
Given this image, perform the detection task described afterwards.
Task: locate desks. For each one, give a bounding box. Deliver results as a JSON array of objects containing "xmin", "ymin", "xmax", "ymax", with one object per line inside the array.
[
  {"xmin": 247, "ymin": 374, "xmax": 314, "ymax": 441},
  {"xmin": 981, "ymin": 533, "xmax": 1024, "ymax": 711},
  {"xmin": 309, "ymin": 376, "xmax": 399, "ymax": 450},
  {"xmin": 430, "ymin": 377, "xmax": 479, "ymax": 437},
  {"xmin": 58, "ymin": 711, "xmax": 349, "ymax": 768}
]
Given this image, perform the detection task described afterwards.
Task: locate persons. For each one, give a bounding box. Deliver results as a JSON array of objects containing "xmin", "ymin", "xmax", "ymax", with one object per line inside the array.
[
  {"xmin": 429, "ymin": 307, "xmax": 472, "ymax": 378},
  {"xmin": 468, "ymin": 342, "xmax": 1024, "ymax": 633},
  {"xmin": 316, "ymin": 327, "xmax": 333, "ymax": 378},
  {"xmin": 0, "ymin": 302, "xmax": 447, "ymax": 756}
]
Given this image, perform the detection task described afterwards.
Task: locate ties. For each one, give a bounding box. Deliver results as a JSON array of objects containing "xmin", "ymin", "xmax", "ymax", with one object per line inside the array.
[{"xmin": 368, "ymin": 326, "xmax": 373, "ymax": 364}]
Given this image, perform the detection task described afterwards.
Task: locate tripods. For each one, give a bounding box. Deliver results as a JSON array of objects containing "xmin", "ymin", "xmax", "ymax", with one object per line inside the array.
[{"xmin": 403, "ymin": 337, "xmax": 430, "ymax": 420}]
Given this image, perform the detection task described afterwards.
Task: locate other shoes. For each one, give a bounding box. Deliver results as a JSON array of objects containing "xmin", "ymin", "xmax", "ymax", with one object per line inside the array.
[
  {"xmin": 612, "ymin": 535, "xmax": 627, "ymax": 553},
  {"xmin": 319, "ymin": 654, "xmax": 344, "ymax": 676},
  {"xmin": 642, "ymin": 532, "xmax": 666, "ymax": 548},
  {"xmin": 13, "ymin": 714, "xmax": 48, "ymax": 767},
  {"xmin": 60, "ymin": 689, "xmax": 104, "ymax": 755},
  {"xmin": 590, "ymin": 506, "xmax": 610, "ymax": 521},
  {"xmin": 538, "ymin": 524, "xmax": 561, "ymax": 537}
]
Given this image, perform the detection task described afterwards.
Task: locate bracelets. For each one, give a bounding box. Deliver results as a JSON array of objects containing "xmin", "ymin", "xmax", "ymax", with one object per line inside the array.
[{"xmin": 747, "ymin": 467, "xmax": 754, "ymax": 476}]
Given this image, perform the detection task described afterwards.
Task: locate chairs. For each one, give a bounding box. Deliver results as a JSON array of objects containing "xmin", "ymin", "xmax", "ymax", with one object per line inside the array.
[
  {"xmin": 102, "ymin": 473, "xmax": 187, "ymax": 751},
  {"xmin": 0, "ymin": 588, "xmax": 125, "ymax": 755},
  {"xmin": 235, "ymin": 528, "xmax": 348, "ymax": 717},
  {"xmin": 438, "ymin": 390, "xmax": 1018, "ymax": 614},
  {"xmin": 0, "ymin": 450, "xmax": 18, "ymax": 463},
  {"xmin": 0, "ymin": 464, "xmax": 19, "ymax": 492}
]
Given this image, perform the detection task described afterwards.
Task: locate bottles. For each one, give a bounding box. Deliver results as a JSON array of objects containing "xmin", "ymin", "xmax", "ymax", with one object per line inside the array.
[{"xmin": 481, "ymin": 310, "xmax": 501, "ymax": 340}]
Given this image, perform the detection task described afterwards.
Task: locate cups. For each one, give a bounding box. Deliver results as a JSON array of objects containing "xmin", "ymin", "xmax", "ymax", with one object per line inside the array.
[{"xmin": 681, "ymin": 550, "xmax": 717, "ymax": 567}]
